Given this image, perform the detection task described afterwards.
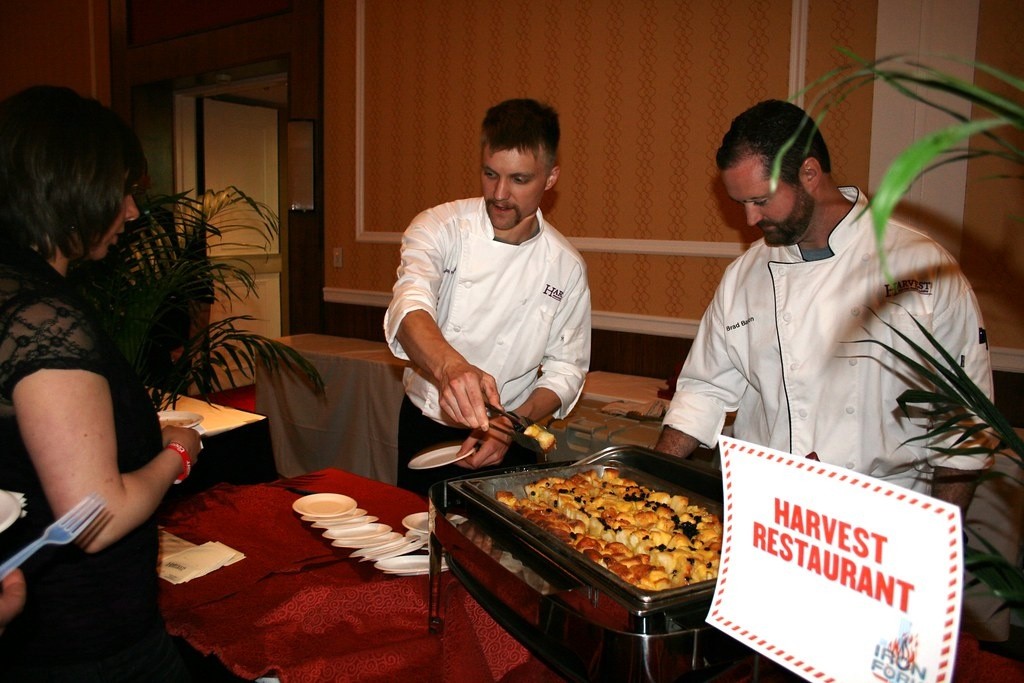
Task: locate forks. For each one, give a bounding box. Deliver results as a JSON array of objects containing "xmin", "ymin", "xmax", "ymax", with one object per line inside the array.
[{"xmin": 0, "ymin": 491, "xmax": 109, "ymax": 582}]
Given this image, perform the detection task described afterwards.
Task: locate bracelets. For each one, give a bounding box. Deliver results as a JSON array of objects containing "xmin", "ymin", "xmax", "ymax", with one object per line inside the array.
[{"xmin": 165, "ymin": 442, "xmax": 192, "ymax": 484}]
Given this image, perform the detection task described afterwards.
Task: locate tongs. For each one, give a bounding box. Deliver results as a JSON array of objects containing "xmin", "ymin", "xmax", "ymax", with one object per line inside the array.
[{"xmin": 484, "ymin": 400, "xmax": 550, "ymax": 454}]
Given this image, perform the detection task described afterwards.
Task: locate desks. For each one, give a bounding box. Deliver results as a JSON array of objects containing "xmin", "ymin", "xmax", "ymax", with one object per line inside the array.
[
  {"xmin": 253, "ymin": 333, "xmax": 714, "ymax": 482},
  {"xmin": 152, "ymin": 468, "xmax": 1024, "ymax": 683}
]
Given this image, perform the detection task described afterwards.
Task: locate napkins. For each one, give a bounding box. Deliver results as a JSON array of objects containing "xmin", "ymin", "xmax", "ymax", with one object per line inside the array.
[{"xmin": 157, "ymin": 529, "xmax": 247, "ymax": 585}]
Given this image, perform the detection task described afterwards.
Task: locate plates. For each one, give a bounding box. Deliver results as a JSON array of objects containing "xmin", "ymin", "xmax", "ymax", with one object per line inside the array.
[
  {"xmin": 408, "ymin": 444, "xmax": 475, "ymax": 470},
  {"xmin": 156, "ymin": 410, "xmax": 203, "ymax": 428},
  {"xmin": 0, "ymin": 489, "xmax": 22, "ymax": 533},
  {"xmin": 292, "ymin": 492, "xmax": 466, "ymax": 576}
]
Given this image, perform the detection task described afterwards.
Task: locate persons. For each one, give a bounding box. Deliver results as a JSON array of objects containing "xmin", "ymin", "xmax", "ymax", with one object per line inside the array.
[
  {"xmin": 653, "ymin": 99, "xmax": 995, "ymax": 526},
  {"xmin": 0, "ymin": 567, "xmax": 26, "ymax": 635},
  {"xmin": 0, "ymin": 84, "xmax": 202, "ymax": 683},
  {"xmin": 383, "ymin": 98, "xmax": 592, "ymax": 495}
]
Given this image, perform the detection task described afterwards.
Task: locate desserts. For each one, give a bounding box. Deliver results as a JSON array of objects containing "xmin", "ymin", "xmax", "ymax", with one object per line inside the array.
[
  {"xmin": 496, "ymin": 468, "xmax": 723, "ymax": 592},
  {"xmin": 524, "ymin": 424, "xmax": 558, "ymax": 452}
]
